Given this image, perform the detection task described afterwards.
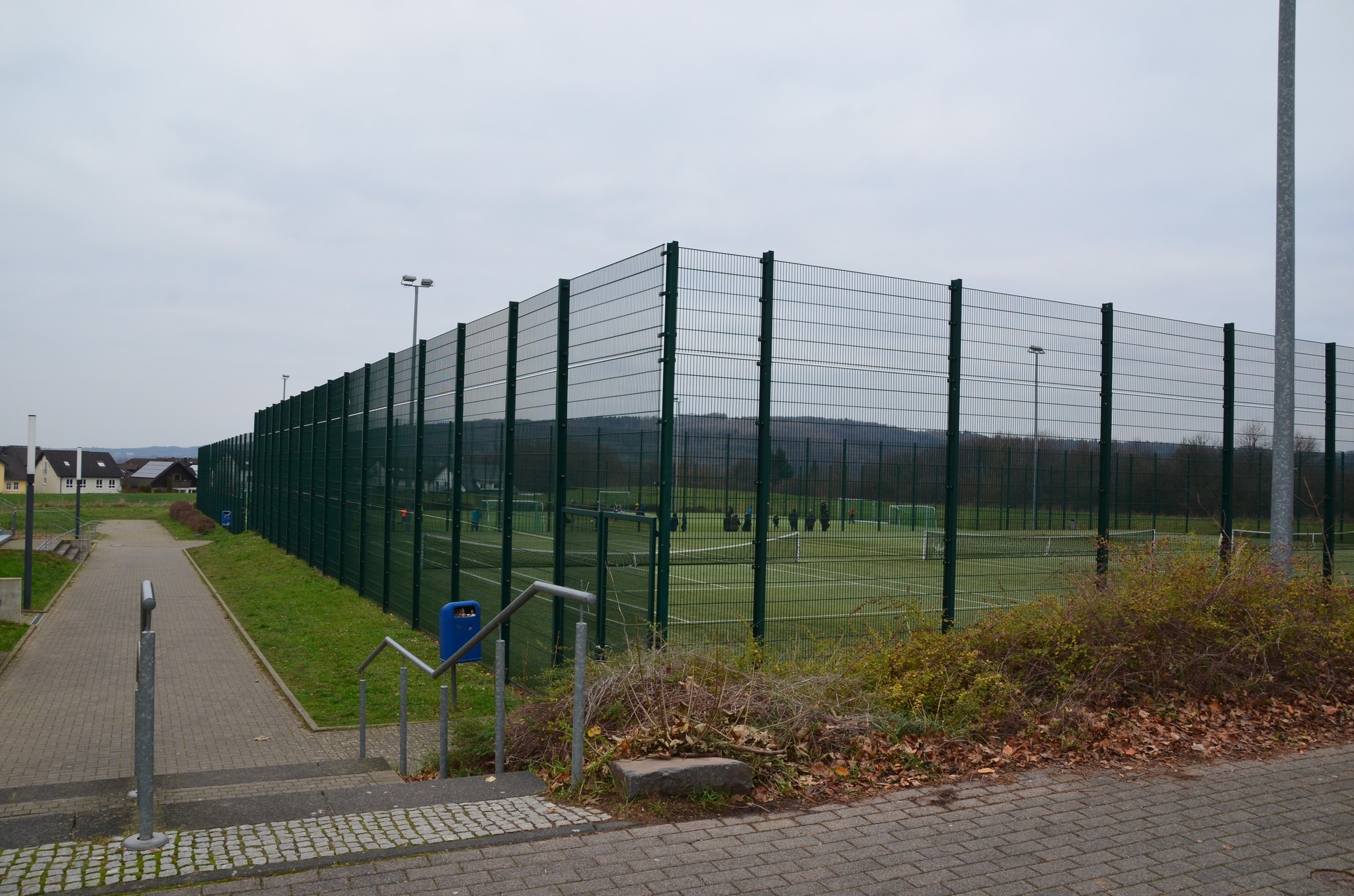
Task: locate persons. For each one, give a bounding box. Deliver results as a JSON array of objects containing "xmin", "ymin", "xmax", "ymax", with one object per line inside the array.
[
  {"xmin": 772, "ymin": 513, "xmax": 779, "ymax": 529},
  {"xmin": 806, "ymin": 509, "xmax": 816, "ymax": 531},
  {"xmin": 789, "ymin": 509, "xmax": 798, "ymax": 531},
  {"xmin": 641, "ymin": 504, "xmax": 646, "ymax": 516},
  {"xmin": 848, "ymin": 506, "xmax": 855, "ymax": 523},
  {"xmin": 347, "ymin": 501, "xmax": 361, "ymax": 516},
  {"xmin": 723, "ymin": 513, "xmax": 733, "ymax": 532},
  {"xmin": 398, "ymin": 509, "xmax": 406, "ymax": 525},
  {"xmin": 819, "ymin": 500, "xmax": 827, "ymax": 520},
  {"xmin": 619, "ymin": 504, "xmax": 623, "ymax": 514},
  {"xmin": 748, "ymin": 506, "xmax": 753, "ymax": 519},
  {"xmin": 571, "ymin": 499, "xmax": 577, "ymax": 523},
  {"xmin": 681, "ymin": 515, "xmax": 687, "ymax": 532},
  {"xmin": 655, "ymin": 505, "xmax": 659, "ymax": 522},
  {"xmin": 470, "ymin": 506, "xmax": 481, "ymax": 532},
  {"xmin": 820, "ymin": 510, "xmax": 830, "ymax": 531},
  {"xmin": 613, "ymin": 501, "xmax": 618, "ymax": 522},
  {"xmin": 635, "ymin": 503, "xmax": 641, "ymax": 515},
  {"xmin": 670, "ymin": 513, "xmax": 679, "ymax": 532},
  {"xmin": 730, "ymin": 514, "xmax": 741, "ymax": 531},
  {"xmin": 742, "ymin": 514, "xmax": 752, "ymax": 532},
  {"xmin": 728, "ymin": 506, "xmax": 734, "ymax": 515}
]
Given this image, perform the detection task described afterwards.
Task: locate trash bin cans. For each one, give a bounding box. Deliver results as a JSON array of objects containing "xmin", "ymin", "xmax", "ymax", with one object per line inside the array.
[
  {"xmin": 221, "ymin": 511, "xmax": 233, "ymax": 529},
  {"xmin": 439, "ymin": 600, "xmax": 482, "ymax": 664}
]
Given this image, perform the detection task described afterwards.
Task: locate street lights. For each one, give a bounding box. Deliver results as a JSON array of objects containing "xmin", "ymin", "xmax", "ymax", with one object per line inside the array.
[
  {"xmin": 1026, "ymin": 345, "xmax": 1046, "ymax": 530},
  {"xmin": 282, "ymin": 374, "xmax": 288, "ymax": 401},
  {"xmin": 399, "ymin": 274, "xmax": 435, "ymax": 512}
]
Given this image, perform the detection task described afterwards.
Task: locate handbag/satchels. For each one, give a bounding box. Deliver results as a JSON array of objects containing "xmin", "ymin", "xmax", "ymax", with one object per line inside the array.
[{"xmin": 827, "ymin": 523, "xmax": 830, "ymax": 529}]
